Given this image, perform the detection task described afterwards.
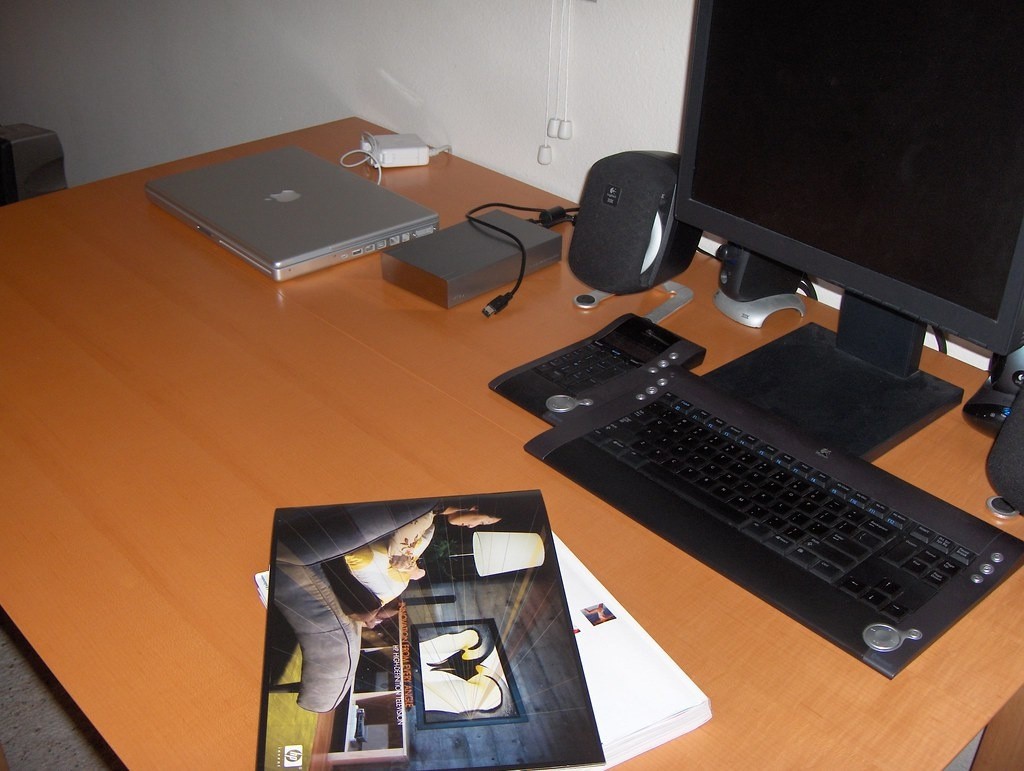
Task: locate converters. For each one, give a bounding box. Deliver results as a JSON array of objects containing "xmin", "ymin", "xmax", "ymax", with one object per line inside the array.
[{"xmin": 361, "ymin": 133, "xmax": 430, "ymax": 167}]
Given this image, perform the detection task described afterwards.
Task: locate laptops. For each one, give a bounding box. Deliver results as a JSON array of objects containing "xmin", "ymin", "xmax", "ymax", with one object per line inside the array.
[{"xmin": 144, "ymin": 143, "xmax": 440, "ymax": 282}]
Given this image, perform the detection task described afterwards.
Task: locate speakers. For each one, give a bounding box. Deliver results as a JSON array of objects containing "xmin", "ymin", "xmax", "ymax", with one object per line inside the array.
[{"xmin": 568, "ymin": 150, "xmax": 702, "ymax": 294}]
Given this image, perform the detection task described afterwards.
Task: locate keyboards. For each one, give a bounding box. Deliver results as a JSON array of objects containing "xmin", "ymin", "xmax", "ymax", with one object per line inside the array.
[{"xmin": 488, "ymin": 310, "xmax": 1024, "ymax": 681}]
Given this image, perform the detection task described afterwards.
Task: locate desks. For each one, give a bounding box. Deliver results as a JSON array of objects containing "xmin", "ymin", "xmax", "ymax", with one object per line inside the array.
[{"xmin": 1, "ymin": 117, "xmax": 1024, "ymax": 771}]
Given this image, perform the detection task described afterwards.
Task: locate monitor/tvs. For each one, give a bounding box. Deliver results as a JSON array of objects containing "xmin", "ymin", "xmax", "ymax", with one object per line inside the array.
[{"xmin": 675, "ymin": 1, "xmax": 1022, "ymax": 355}]
